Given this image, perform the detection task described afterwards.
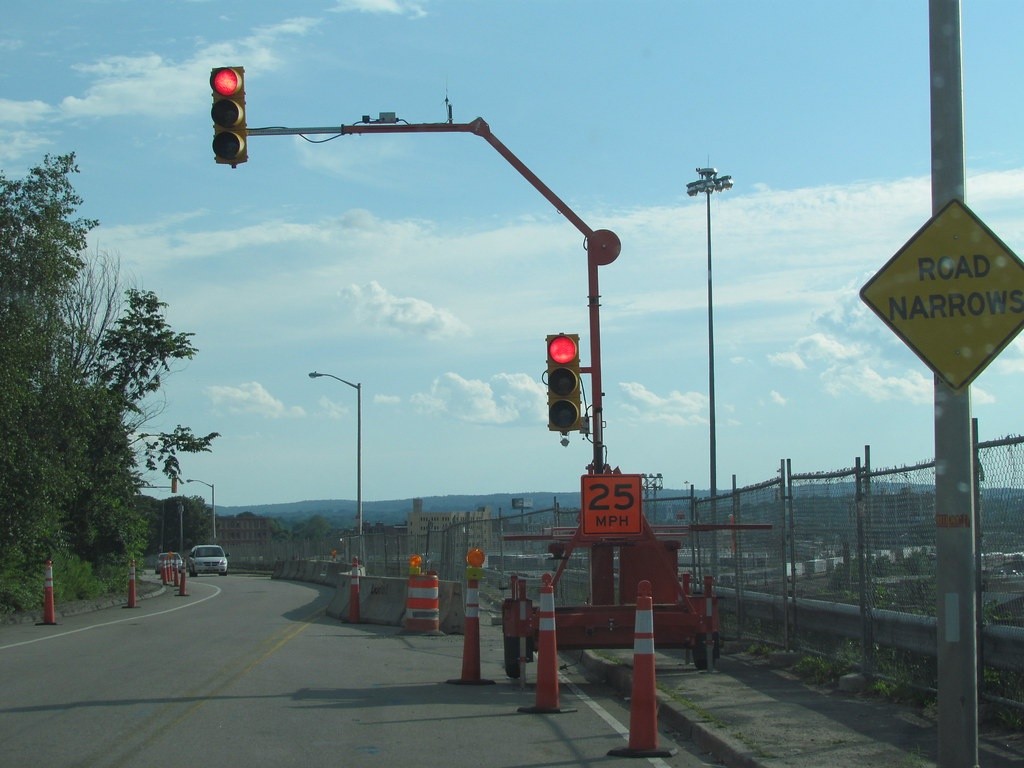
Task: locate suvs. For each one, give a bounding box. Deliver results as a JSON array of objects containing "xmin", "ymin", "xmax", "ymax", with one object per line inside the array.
[{"xmin": 188, "ymin": 545, "xmax": 230, "ymax": 577}]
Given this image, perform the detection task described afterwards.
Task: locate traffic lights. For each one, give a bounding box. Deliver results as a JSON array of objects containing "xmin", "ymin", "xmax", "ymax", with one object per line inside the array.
[
  {"xmin": 546, "ymin": 334, "xmax": 587, "ymax": 435},
  {"xmin": 211, "ymin": 65, "xmax": 248, "ymax": 172}
]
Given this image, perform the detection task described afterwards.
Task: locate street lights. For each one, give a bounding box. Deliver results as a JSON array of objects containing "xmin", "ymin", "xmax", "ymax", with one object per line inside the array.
[
  {"xmin": 308, "ymin": 371, "xmax": 364, "ymax": 562},
  {"xmin": 186, "ymin": 479, "xmax": 217, "ymax": 541},
  {"xmin": 687, "ymin": 165, "xmax": 735, "ymax": 591}
]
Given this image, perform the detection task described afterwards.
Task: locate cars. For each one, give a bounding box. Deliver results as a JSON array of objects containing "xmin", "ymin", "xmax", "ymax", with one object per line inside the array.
[{"xmin": 154, "ymin": 552, "xmax": 182, "ymax": 573}]
